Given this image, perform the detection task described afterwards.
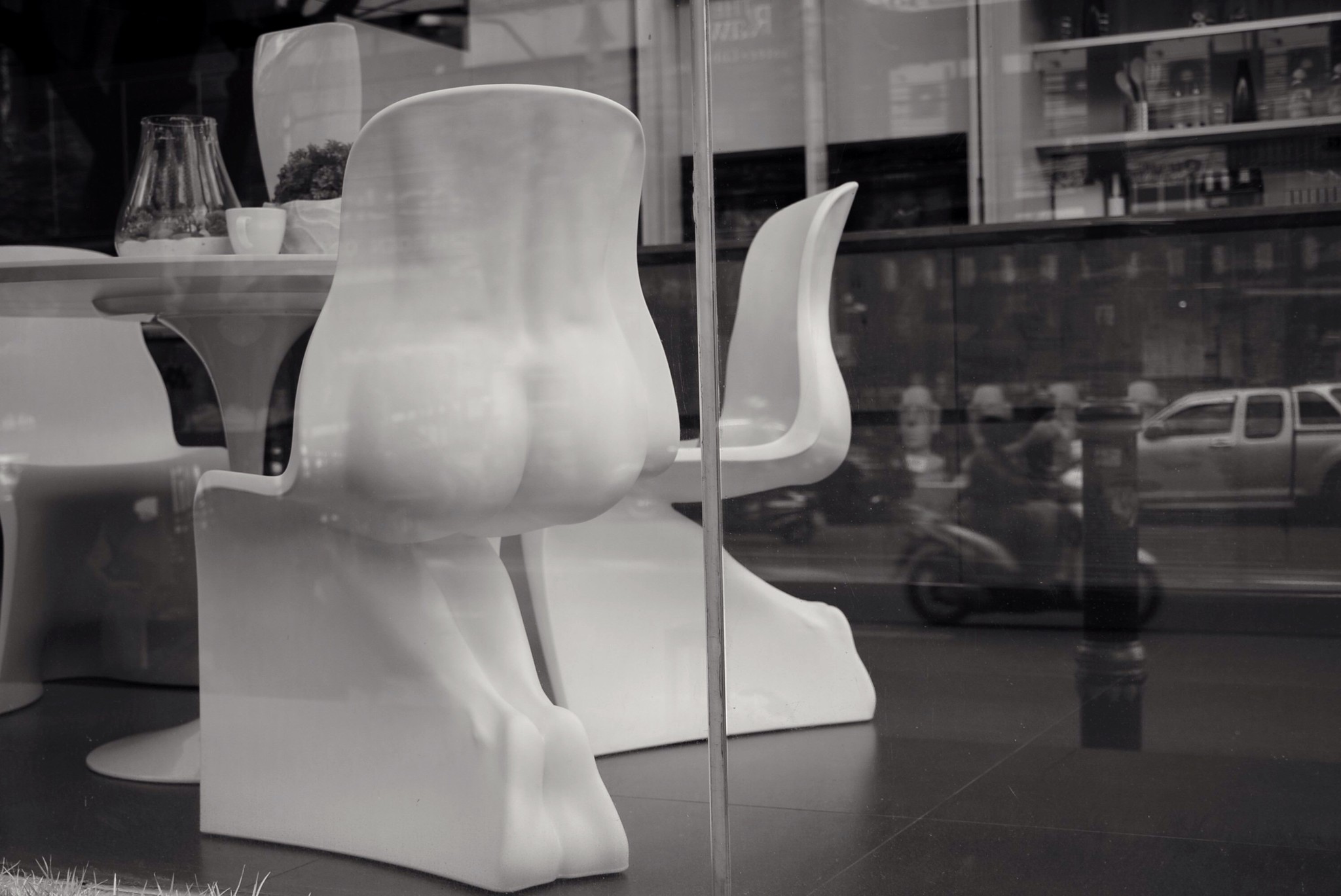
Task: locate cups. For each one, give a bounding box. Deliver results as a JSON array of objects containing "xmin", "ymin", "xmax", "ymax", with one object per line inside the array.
[
  {"xmin": 1127, "ymin": 101, "xmax": 1150, "ymax": 132},
  {"xmin": 225, "ymin": 207, "xmax": 286, "ymax": 255}
]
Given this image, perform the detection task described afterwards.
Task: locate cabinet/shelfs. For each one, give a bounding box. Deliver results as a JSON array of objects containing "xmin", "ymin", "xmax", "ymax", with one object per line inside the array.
[{"xmin": 1029, "ymin": 9, "xmax": 1341, "ymax": 148}]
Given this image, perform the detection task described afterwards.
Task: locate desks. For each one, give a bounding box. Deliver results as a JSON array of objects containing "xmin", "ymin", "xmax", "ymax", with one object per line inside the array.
[{"xmin": 2, "ymin": 256, "xmax": 339, "ymax": 782}]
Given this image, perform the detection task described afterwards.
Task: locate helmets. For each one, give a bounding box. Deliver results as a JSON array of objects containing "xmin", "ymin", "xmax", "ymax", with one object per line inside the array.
[{"xmin": 1012, "ymin": 388, "xmax": 1054, "ymax": 411}]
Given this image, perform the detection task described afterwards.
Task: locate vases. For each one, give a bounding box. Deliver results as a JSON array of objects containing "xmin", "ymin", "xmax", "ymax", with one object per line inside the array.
[{"xmin": 279, "ymin": 197, "xmax": 339, "ymax": 255}]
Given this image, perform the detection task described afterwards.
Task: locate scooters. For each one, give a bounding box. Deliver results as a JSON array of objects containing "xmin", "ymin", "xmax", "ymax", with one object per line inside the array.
[{"xmin": 898, "ymin": 500, "xmax": 1172, "ymax": 647}]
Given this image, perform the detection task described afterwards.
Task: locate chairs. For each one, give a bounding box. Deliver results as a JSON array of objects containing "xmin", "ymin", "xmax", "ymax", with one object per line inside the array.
[
  {"xmin": 0, "ymin": 246, "xmax": 235, "ymax": 715},
  {"xmin": 195, "ymin": 83, "xmax": 681, "ymax": 892},
  {"xmin": 507, "ymin": 180, "xmax": 877, "ymax": 755}
]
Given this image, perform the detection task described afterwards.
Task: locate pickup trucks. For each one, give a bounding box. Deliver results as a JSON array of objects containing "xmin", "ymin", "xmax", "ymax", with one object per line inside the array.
[{"xmin": 1058, "ymin": 384, "xmax": 1339, "ymax": 519}]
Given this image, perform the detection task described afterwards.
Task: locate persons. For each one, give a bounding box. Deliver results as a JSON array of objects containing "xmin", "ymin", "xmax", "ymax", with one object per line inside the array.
[
  {"xmin": 898, "ymin": 380, "xmax": 1168, "ymax": 482},
  {"xmin": 1085, "ymin": 78, "xmax": 1137, "ymax": 218},
  {"xmin": 961, "ymin": 393, "xmax": 1084, "ymax": 579}
]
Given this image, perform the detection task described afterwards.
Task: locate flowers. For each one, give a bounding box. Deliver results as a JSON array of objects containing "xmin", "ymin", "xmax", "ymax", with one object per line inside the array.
[{"xmin": 272, "ymin": 138, "xmax": 354, "ymax": 201}]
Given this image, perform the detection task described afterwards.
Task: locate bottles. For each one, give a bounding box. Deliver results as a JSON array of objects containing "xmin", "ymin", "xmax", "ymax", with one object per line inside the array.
[
  {"xmin": 113, "ymin": 114, "xmax": 244, "ymax": 255},
  {"xmin": 1232, "ymin": 58, "xmax": 1258, "ymax": 123}
]
